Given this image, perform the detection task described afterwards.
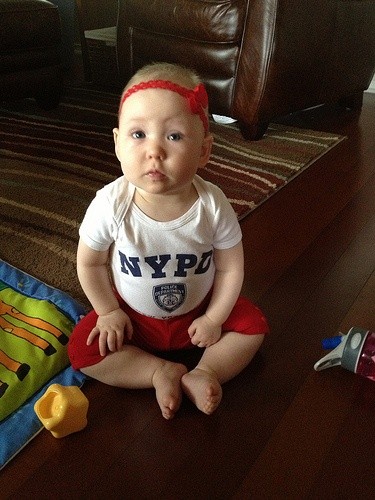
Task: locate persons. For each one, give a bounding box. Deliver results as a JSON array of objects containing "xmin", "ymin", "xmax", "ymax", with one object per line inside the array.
[{"xmin": 68, "ymin": 62, "xmax": 270, "ymax": 419}]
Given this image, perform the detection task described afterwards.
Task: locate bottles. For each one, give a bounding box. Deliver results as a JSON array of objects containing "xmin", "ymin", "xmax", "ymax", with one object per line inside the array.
[{"xmin": 314, "ymin": 327, "xmax": 375, "ymax": 382}]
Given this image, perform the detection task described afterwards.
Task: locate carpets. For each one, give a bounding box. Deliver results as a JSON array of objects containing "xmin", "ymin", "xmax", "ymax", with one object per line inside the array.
[{"xmin": 0, "ymin": 84, "xmax": 349, "ymax": 309}]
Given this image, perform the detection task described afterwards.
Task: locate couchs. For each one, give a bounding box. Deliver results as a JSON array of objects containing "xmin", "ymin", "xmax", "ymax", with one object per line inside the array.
[
  {"xmin": 116, "ymin": 0, "xmax": 375, "ymax": 142},
  {"xmin": 0, "ymin": 0, "xmax": 64, "ymax": 109}
]
